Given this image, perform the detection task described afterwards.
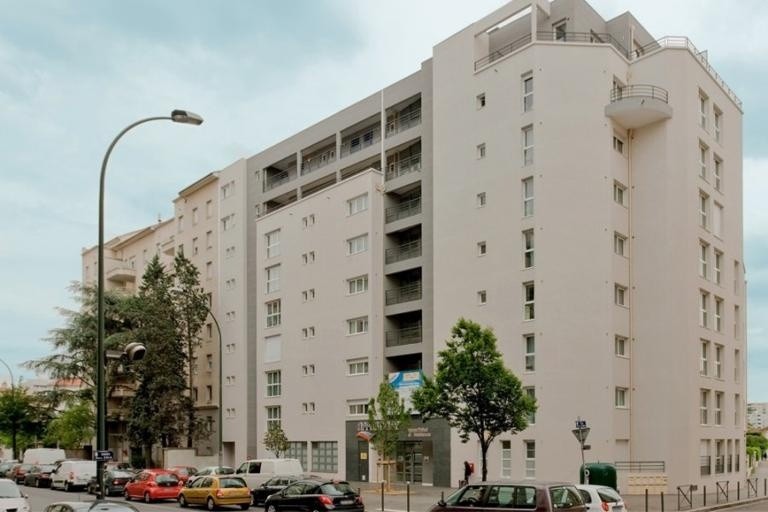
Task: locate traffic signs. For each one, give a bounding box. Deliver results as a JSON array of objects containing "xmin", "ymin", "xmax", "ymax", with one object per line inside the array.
[{"xmin": 92, "ymin": 450, "xmax": 114, "ymax": 462}]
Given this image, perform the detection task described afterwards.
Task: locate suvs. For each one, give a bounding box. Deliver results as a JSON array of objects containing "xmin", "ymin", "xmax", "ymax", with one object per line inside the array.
[{"xmin": 423, "ymin": 478, "xmax": 586, "ymax": 512}]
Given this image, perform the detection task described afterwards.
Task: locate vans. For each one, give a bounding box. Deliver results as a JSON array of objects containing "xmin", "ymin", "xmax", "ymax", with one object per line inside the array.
[
  {"xmin": 228, "ymin": 457, "xmax": 304, "ymax": 488},
  {"xmin": 21, "ymin": 447, "xmax": 67, "ymax": 465}
]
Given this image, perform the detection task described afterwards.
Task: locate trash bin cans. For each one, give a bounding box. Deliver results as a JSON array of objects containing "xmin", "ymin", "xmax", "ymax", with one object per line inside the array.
[{"xmin": 580, "ymin": 463, "xmax": 617, "ymax": 491}]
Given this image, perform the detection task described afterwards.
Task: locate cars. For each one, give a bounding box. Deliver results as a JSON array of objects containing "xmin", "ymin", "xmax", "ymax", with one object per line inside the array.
[
  {"xmin": 43, "ymin": 500, "xmax": 139, "ymax": 512},
  {"xmin": 0, "ymin": 458, "xmax": 235, "ymax": 498},
  {"xmin": 526, "ymin": 483, "xmax": 627, "ymax": 512},
  {"xmin": 121, "ymin": 469, "xmax": 185, "ymax": 504},
  {"xmin": 175, "ymin": 475, "xmax": 252, "ymax": 512},
  {"xmin": 250, "ymin": 472, "xmax": 322, "ymax": 506},
  {"xmin": 0, "ymin": 479, "xmax": 31, "ymax": 512},
  {"xmin": 262, "ymin": 477, "xmax": 365, "ymax": 511}
]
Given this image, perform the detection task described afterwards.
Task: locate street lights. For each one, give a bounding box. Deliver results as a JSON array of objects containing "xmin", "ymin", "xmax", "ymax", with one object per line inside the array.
[
  {"xmin": 92, "ymin": 109, "xmax": 203, "ymax": 499},
  {"xmin": 166, "ymin": 285, "xmax": 225, "ymax": 469}
]
